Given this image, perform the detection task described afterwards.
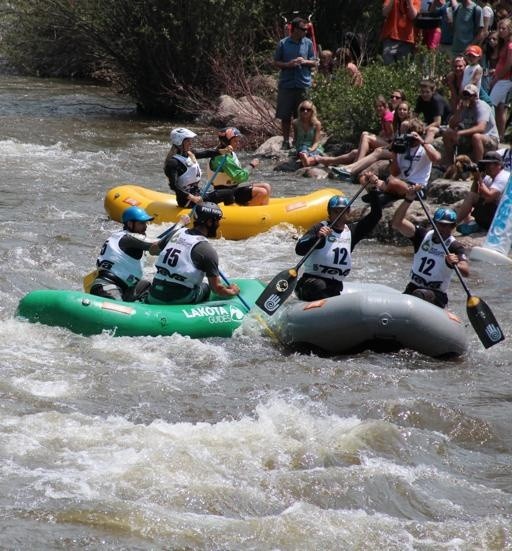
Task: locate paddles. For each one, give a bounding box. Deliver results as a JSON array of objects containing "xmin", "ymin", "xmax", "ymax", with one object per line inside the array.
[
  {"xmin": 416, "ymin": 188, "xmax": 505, "ymax": 350},
  {"xmin": 254, "ymin": 180, "xmax": 370, "ymax": 316},
  {"xmin": 217, "ymin": 270, "xmax": 279, "ymax": 344},
  {"xmin": 83, "ymin": 222, "xmax": 179, "ymax": 294}
]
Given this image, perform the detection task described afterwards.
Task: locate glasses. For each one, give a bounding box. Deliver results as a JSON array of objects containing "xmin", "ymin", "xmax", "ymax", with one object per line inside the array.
[
  {"xmin": 297, "ymin": 26, "xmax": 308, "ymax": 32},
  {"xmin": 300, "ymin": 107, "xmax": 312, "ymax": 112},
  {"xmin": 391, "ymin": 95, "xmax": 402, "ymax": 99}
]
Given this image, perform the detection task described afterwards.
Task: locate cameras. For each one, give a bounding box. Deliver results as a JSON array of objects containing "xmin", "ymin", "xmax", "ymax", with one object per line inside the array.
[{"xmin": 468, "ymin": 164, "xmax": 486, "ymax": 176}]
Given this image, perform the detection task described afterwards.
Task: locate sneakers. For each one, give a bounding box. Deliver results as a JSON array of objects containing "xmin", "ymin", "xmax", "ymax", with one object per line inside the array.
[{"xmin": 281, "ymin": 142, "xmax": 290, "ymax": 149}]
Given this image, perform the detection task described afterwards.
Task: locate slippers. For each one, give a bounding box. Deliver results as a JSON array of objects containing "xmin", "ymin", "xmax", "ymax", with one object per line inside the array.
[{"xmin": 332, "ymin": 167, "xmax": 352, "ymax": 178}]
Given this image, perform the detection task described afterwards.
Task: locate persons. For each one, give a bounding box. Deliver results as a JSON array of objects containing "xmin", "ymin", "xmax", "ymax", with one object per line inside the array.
[
  {"xmin": 205, "ymin": 127, "xmax": 272, "ymax": 210},
  {"xmin": 86, "ymin": 204, "xmax": 191, "ymax": 309},
  {"xmin": 162, "ymin": 127, "xmax": 241, "ymax": 210},
  {"xmin": 291, "ymin": 172, "xmax": 383, "ymax": 303},
  {"xmin": 388, "ymin": 184, "xmax": 469, "ymax": 308},
  {"xmin": 269, "ymin": 1, "xmax": 511, "ymax": 237},
  {"xmin": 147, "ymin": 203, "xmax": 242, "ymax": 306}
]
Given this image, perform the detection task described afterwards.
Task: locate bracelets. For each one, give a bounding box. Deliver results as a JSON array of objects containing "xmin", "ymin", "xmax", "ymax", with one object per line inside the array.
[
  {"xmin": 248, "ymin": 163, "xmax": 256, "ymax": 169},
  {"xmin": 403, "ymin": 197, "xmax": 414, "ymax": 204}
]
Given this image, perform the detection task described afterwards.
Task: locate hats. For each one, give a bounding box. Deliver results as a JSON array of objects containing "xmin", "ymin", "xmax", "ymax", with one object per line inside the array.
[
  {"xmin": 477, "ymin": 151, "xmax": 502, "ymax": 163},
  {"xmin": 464, "ymin": 44, "xmax": 482, "ymax": 57},
  {"xmin": 462, "ymin": 83, "xmax": 479, "ymax": 98}
]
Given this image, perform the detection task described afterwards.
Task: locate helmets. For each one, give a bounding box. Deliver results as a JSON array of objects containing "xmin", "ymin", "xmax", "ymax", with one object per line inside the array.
[
  {"xmin": 193, "ymin": 202, "xmax": 222, "ymax": 220},
  {"xmin": 434, "ymin": 207, "xmax": 457, "ymax": 224},
  {"xmin": 218, "ymin": 127, "xmax": 242, "ymax": 145},
  {"xmin": 327, "ymin": 195, "xmax": 350, "ymax": 215},
  {"xmin": 122, "ymin": 206, "xmax": 154, "ymax": 223},
  {"xmin": 170, "ymin": 127, "xmax": 197, "ymax": 146}
]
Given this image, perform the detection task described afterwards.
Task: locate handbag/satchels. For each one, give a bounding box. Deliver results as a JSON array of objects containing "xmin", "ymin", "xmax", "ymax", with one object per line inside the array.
[{"xmin": 414, "ymin": 12, "xmax": 442, "ymax": 28}]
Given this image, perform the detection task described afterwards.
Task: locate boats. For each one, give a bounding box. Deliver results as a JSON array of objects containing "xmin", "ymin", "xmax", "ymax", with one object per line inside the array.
[
  {"xmin": 14, "ymin": 276, "xmax": 270, "ymax": 340},
  {"xmin": 104, "ymin": 185, "xmax": 348, "ymax": 242},
  {"xmin": 266, "ymin": 280, "xmax": 471, "ymax": 362}
]
